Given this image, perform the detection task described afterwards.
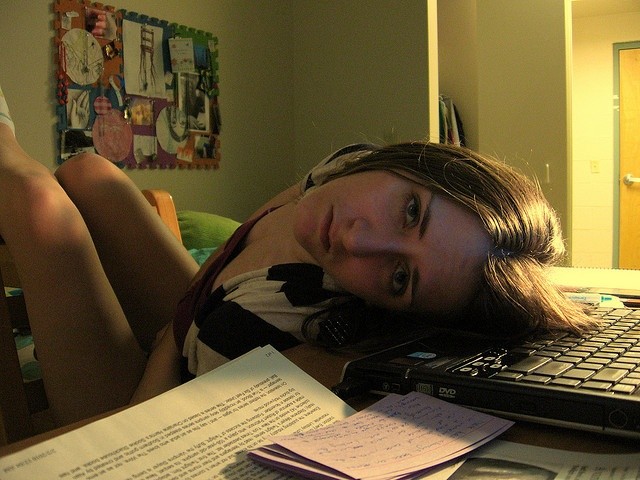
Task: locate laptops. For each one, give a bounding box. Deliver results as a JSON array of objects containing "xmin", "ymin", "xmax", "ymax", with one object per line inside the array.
[{"xmin": 333, "ymin": 307, "xmax": 640, "ymax": 439}]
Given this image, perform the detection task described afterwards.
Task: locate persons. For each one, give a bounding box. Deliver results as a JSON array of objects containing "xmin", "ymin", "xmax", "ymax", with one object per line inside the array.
[
  {"xmin": 71, "ymin": 92, "xmax": 92, "ymax": 130},
  {"xmin": 0, "ymin": 87, "xmax": 600, "ymax": 429}
]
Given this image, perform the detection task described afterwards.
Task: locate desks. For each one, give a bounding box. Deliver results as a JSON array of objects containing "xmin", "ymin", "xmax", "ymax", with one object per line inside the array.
[{"xmin": 0, "ymin": 344, "xmax": 639, "ymax": 476}]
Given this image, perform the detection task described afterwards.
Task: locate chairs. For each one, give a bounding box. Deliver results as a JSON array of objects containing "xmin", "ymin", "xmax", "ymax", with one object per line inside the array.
[{"xmin": 1, "ymin": 188, "xmax": 184, "ymax": 444}]
